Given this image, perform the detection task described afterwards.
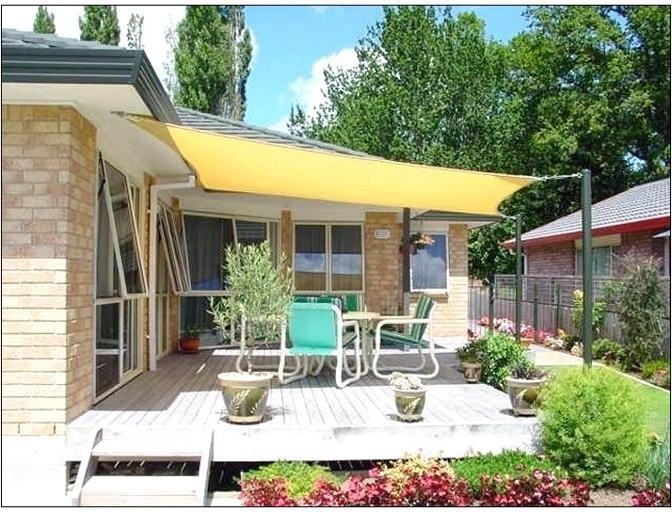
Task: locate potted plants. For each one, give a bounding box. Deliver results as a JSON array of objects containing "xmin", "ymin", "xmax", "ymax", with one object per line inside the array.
[
  {"xmin": 175, "ymin": 321, "xmax": 206, "ymax": 354},
  {"xmin": 409, "ymin": 232, "xmax": 437, "ymax": 255},
  {"xmin": 203, "ymin": 239, "xmax": 296, "ymax": 423},
  {"xmin": 454, "ymin": 340, "xmax": 485, "ymax": 384},
  {"xmin": 385, "ymin": 370, "xmax": 427, "ymax": 421},
  {"xmin": 505, "ymin": 356, "xmax": 549, "ymax": 417}
]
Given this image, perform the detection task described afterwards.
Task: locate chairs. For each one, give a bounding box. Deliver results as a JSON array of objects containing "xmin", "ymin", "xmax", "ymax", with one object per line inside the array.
[{"xmin": 234, "ymin": 291, "xmax": 440, "ymax": 388}]
